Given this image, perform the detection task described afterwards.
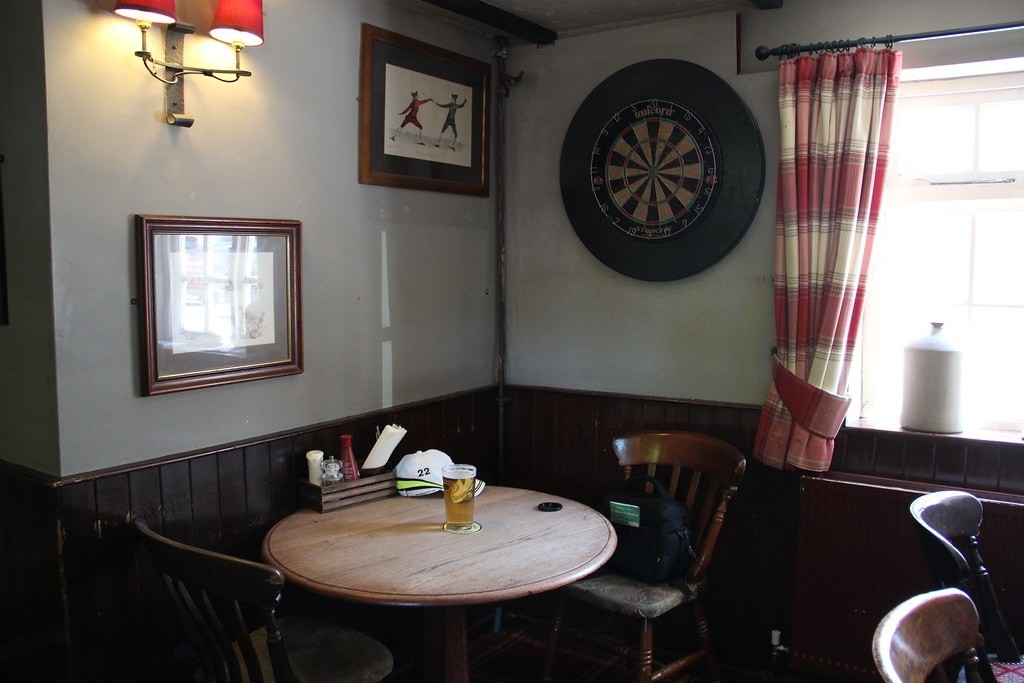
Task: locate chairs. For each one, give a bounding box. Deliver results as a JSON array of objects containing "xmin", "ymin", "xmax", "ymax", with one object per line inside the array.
[
  {"xmin": 538, "ymin": 430, "xmax": 747, "ymax": 683},
  {"xmin": 872, "ymin": 586, "xmax": 985, "ymax": 683},
  {"xmin": 908, "ymin": 490, "xmax": 1024, "ymax": 683},
  {"xmin": 132, "ymin": 515, "xmax": 393, "ymax": 683}
]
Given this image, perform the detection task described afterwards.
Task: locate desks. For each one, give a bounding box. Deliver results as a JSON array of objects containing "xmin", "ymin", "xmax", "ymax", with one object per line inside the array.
[{"xmin": 262, "ymin": 486, "xmax": 618, "ymax": 683}]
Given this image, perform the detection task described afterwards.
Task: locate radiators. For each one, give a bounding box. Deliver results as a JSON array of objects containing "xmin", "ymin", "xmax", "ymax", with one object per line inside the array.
[{"xmin": 769, "ymin": 471, "xmax": 1024, "ymax": 683}]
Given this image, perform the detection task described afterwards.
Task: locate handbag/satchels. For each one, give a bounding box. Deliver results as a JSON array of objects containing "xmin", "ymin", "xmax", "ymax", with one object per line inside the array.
[{"xmin": 599, "ymin": 475, "xmax": 699, "ymax": 597}]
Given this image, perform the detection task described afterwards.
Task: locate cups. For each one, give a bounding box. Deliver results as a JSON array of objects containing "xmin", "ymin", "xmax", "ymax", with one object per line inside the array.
[{"xmin": 442, "ymin": 464, "xmax": 477, "ymax": 530}]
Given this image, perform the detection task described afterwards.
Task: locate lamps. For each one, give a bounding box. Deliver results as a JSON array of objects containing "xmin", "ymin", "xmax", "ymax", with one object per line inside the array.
[{"xmin": 112, "ymin": 0, "xmax": 265, "ymax": 128}]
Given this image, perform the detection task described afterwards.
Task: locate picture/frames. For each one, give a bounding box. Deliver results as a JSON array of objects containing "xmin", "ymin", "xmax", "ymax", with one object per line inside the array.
[
  {"xmin": 135, "ymin": 213, "xmax": 304, "ymax": 395},
  {"xmin": 359, "ymin": 22, "xmax": 493, "ymax": 198}
]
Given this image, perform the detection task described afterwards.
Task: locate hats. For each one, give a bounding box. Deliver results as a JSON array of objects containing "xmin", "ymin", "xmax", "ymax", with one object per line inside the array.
[{"xmin": 392, "ymin": 449, "xmax": 486, "ymax": 499}]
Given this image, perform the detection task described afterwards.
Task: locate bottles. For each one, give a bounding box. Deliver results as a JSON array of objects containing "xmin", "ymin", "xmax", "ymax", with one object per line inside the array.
[
  {"xmin": 320, "ymin": 456, "xmax": 344, "ymax": 486},
  {"xmin": 306, "ymin": 449, "xmax": 324, "ymax": 486},
  {"xmin": 900, "ymin": 320, "xmax": 965, "ymax": 434},
  {"xmin": 339, "ymin": 434, "xmax": 360, "ymax": 482}
]
